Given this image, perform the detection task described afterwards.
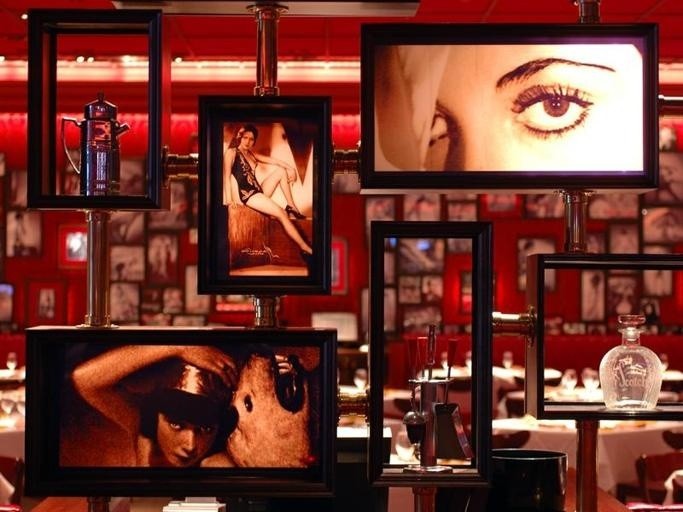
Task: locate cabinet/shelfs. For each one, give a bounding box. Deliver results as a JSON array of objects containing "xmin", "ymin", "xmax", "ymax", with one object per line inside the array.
[
  {"xmin": 524, "ymin": 253, "xmax": 682, "ymax": 422},
  {"xmin": 26, "ymin": 6, "xmax": 164, "ymax": 208}
]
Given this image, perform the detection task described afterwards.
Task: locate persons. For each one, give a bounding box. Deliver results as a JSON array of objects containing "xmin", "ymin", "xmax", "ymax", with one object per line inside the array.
[
  {"xmin": 222, "ymin": 120, "xmax": 313, "ymax": 269},
  {"xmin": 423, "ymin": 278, "xmax": 441, "ymax": 302},
  {"xmin": 642, "ymin": 299, "xmax": 659, "ymax": 324},
  {"xmin": 12, "ymin": 213, "xmax": 38, "ymax": 256},
  {"xmin": 72, "ymin": 343, "xmax": 241, "ymax": 468},
  {"xmin": 375, "ymin": 45, "xmax": 644, "ymax": 172}
]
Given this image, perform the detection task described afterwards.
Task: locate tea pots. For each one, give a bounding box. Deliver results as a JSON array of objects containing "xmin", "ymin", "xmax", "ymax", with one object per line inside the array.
[{"xmin": 59, "ymin": 89, "xmax": 130, "ymax": 193}]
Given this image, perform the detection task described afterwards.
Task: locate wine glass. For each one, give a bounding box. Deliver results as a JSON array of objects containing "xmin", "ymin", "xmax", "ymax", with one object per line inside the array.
[
  {"xmin": 0, "ymin": 351, "xmax": 26, "ymax": 420},
  {"xmin": 352, "ymin": 366, "xmax": 368, "ymax": 392},
  {"xmin": 557, "ymin": 368, "xmax": 601, "ymax": 400},
  {"xmin": 420, "ymin": 348, "xmax": 518, "ymax": 376}
]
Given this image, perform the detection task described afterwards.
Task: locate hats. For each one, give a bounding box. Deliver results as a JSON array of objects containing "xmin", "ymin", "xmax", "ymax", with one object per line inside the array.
[{"xmin": 169, "ymin": 362, "xmax": 231, "ymax": 405}]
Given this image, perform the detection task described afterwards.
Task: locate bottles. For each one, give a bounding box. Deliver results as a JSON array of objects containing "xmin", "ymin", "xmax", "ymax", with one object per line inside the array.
[{"xmin": 593, "ymin": 310, "xmax": 665, "ymax": 411}]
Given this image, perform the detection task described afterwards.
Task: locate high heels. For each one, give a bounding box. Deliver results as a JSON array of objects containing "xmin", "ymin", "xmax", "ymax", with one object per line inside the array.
[
  {"xmin": 285, "ymin": 205, "xmax": 306, "ymax": 219},
  {"xmin": 300, "ymin": 250, "xmax": 312, "ymax": 267}
]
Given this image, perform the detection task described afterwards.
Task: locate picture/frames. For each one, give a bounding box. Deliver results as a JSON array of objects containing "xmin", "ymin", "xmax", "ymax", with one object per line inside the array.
[
  {"xmin": 104, "ymin": 161, "xmax": 210, "ymax": 327},
  {"xmin": 196, "ymin": 91, "xmax": 336, "ymax": 296},
  {"xmin": 1, "ymin": 281, "xmax": 16, "ymax": 326},
  {"xmin": 57, "ymin": 222, "xmax": 87, "ymax": 273},
  {"xmin": 9, "ymin": 170, "xmax": 30, "ymax": 210},
  {"xmin": 356, "ymin": 20, "xmax": 663, "ymax": 193},
  {"xmin": 7, "ymin": 211, "xmax": 44, "ymax": 258},
  {"xmin": 0, "ymin": 154, "xmax": 7, "ymax": 177},
  {"xmin": 22, "ymin": 325, "xmax": 338, "ymax": 500},
  {"xmin": 25, "ymin": 276, "xmax": 67, "ymax": 326}
]
